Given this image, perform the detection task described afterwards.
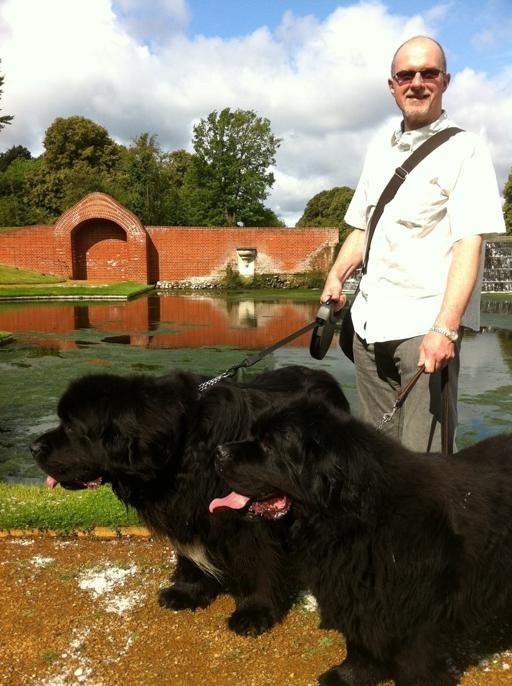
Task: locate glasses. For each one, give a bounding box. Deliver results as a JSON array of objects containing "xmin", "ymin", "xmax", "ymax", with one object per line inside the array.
[{"xmin": 394, "ymin": 68, "xmax": 445, "ymax": 83}]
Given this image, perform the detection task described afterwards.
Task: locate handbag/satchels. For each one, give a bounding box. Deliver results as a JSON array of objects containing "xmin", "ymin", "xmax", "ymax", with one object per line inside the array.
[{"xmin": 340, "ymin": 291, "xmax": 354, "ymax": 360}]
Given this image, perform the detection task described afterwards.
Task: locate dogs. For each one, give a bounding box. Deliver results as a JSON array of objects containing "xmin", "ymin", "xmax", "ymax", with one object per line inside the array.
[
  {"xmin": 209, "ymin": 391, "xmax": 512, "ymax": 686},
  {"xmin": 28, "ymin": 365, "xmax": 350, "ymax": 639}
]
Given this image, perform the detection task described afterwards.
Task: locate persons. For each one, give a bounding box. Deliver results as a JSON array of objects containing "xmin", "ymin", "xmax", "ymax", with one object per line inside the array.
[{"xmin": 319, "ymin": 37, "xmax": 506, "ymax": 456}]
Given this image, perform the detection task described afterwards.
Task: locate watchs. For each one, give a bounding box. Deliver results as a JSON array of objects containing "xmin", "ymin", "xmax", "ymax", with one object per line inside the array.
[{"xmin": 429, "ymin": 327, "xmax": 460, "ymax": 345}]
